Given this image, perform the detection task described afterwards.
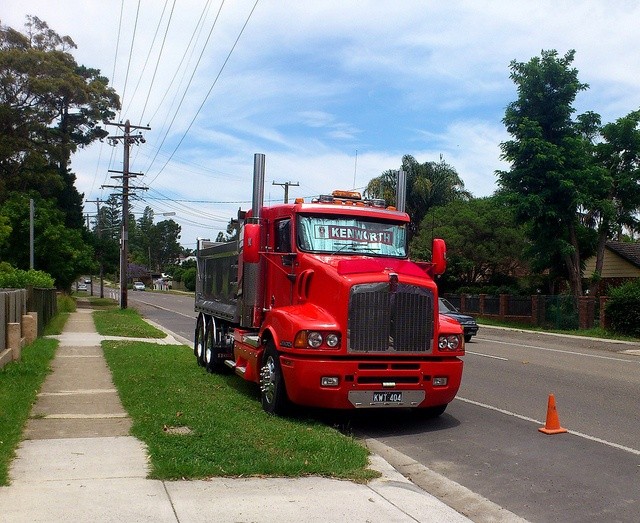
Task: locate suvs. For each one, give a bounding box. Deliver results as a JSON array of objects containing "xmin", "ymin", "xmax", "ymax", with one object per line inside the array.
[
  {"xmin": 438, "ymin": 298, "xmax": 478, "ymax": 343},
  {"xmin": 133, "ymin": 282, "xmax": 145, "ymax": 291}
]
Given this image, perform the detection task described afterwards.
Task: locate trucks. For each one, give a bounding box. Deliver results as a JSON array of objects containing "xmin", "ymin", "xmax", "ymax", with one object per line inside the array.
[{"xmin": 195, "ymin": 154, "xmax": 465, "ymax": 422}]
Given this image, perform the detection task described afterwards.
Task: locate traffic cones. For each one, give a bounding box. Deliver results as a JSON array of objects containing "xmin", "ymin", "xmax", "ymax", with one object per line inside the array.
[{"xmin": 538, "ymin": 394, "xmax": 567, "ymax": 435}]
